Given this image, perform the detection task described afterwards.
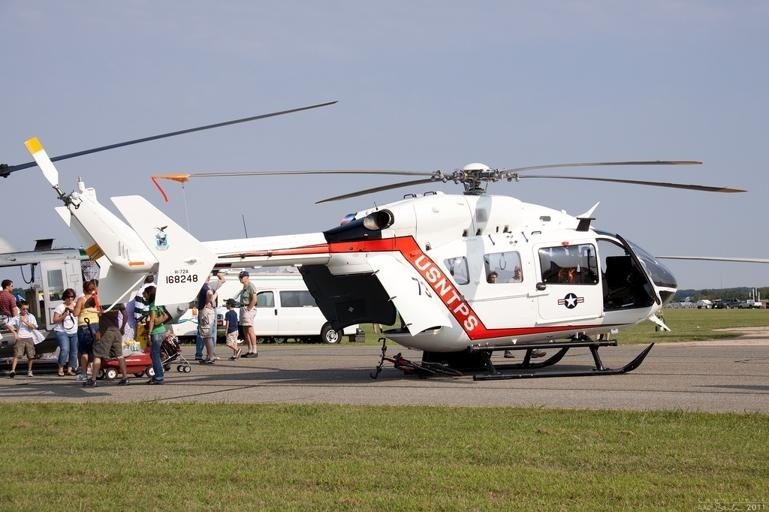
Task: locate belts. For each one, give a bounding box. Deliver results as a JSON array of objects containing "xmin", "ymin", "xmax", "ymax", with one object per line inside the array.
[{"xmin": 239, "ymin": 303, "xmax": 252, "ymax": 308}]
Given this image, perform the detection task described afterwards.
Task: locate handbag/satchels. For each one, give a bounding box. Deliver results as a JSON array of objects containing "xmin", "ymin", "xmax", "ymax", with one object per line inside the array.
[{"xmin": 154, "ymin": 311, "xmax": 169, "ymax": 326}]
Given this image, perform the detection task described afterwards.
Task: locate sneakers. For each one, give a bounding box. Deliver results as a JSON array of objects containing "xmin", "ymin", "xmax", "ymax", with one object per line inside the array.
[
  {"xmin": 229, "ymin": 348, "xmax": 259, "ymax": 360},
  {"xmin": 195, "ymin": 348, "xmax": 216, "ymax": 365},
  {"xmin": 28, "ymin": 370, "xmax": 33, "ymax": 377},
  {"xmin": 117, "ymin": 378, "xmax": 129, "ymax": 385},
  {"xmin": 9, "ymin": 370, "xmax": 16, "ymax": 377},
  {"xmin": 147, "ymin": 377, "xmax": 165, "ymax": 385},
  {"xmin": 83, "ymin": 377, "xmax": 97, "ymax": 387}
]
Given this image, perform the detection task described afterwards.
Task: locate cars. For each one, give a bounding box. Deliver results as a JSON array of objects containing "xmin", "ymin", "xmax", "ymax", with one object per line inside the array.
[{"xmin": 665, "ymin": 298, "xmax": 763, "ymax": 309}]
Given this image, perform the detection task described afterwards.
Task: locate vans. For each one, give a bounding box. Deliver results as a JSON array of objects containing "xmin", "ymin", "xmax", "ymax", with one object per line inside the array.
[{"xmin": 217, "ymin": 286, "xmax": 362, "ymax": 344}]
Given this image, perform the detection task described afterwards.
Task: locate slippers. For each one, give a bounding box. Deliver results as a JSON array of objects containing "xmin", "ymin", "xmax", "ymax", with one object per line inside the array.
[
  {"xmin": 66, "ymin": 372, "xmax": 76, "ymax": 375},
  {"xmin": 58, "ymin": 372, "xmax": 65, "ymax": 376}
]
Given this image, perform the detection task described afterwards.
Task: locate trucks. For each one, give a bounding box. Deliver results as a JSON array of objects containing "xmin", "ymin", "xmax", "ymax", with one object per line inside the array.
[
  {"xmin": 163, "ymin": 272, "xmax": 307, "ymax": 346},
  {"xmin": 0, "ymin": 239, "xmax": 101, "ymax": 365}
]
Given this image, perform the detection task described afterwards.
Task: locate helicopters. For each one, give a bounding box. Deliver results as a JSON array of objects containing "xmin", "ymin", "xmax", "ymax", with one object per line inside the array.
[
  {"xmin": 0, "ymin": 100, "xmax": 342, "ymax": 178},
  {"xmin": 110, "ymin": 161, "xmax": 748, "ymax": 382}
]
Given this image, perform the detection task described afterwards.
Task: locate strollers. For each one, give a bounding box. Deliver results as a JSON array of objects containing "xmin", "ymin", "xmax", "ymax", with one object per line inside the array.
[{"xmin": 162, "ymin": 331, "xmax": 192, "ymax": 374}]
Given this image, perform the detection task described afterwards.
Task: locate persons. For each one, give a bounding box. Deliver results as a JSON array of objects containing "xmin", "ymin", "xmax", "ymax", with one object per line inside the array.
[
  {"xmin": 222, "ymin": 298, "xmax": 243, "ymax": 360},
  {"xmin": 52, "ymin": 287, "xmax": 79, "ymax": 378},
  {"xmin": 0, "ymin": 278, "xmax": 18, "ymax": 330},
  {"xmin": 5, "ymin": 299, "xmax": 39, "ymax": 378},
  {"xmin": 531, "ymin": 348, "xmax": 547, "ymax": 359},
  {"xmin": 72, "ymin": 278, "xmax": 131, "ymax": 387},
  {"xmin": 141, "ymin": 285, "xmax": 168, "ymax": 384},
  {"xmin": 194, "ymin": 269, "xmax": 226, "ymax": 365},
  {"xmin": 501, "ymin": 349, "xmax": 516, "ymax": 359},
  {"xmin": 234, "ymin": 270, "xmax": 258, "ymax": 359},
  {"xmin": 134, "ymin": 274, "xmax": 156, "ymax": 325},
  {"xmin": 487, "ymin": 271, "xmax": 498, "ymax": 283},
  {"xmin": 506, "ymin": 265, "xmax": 522, "ymax": 283}
]
